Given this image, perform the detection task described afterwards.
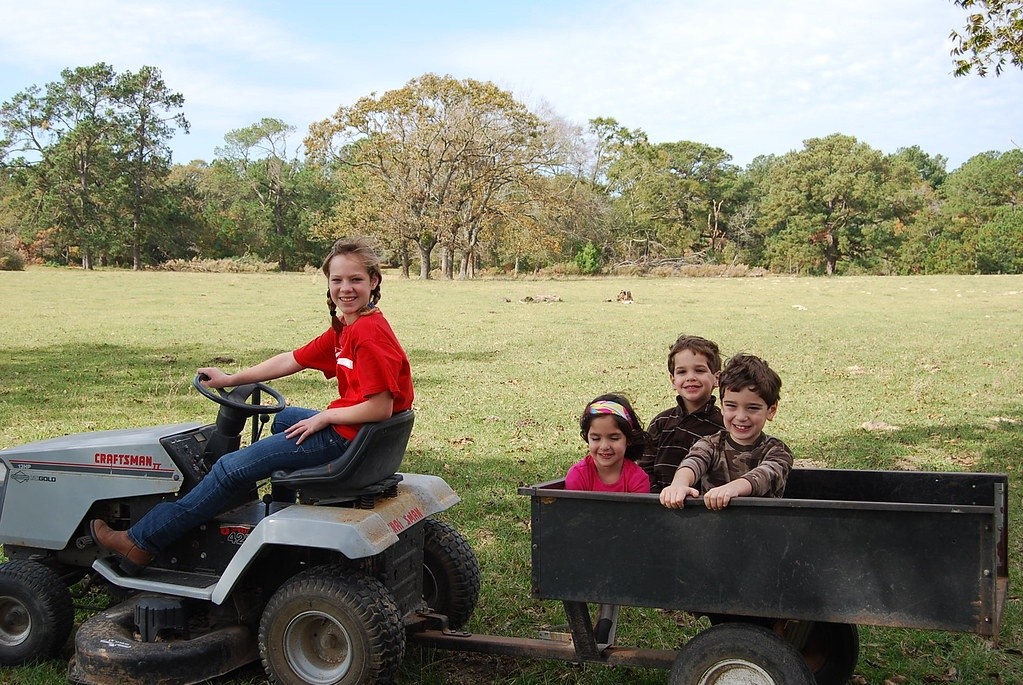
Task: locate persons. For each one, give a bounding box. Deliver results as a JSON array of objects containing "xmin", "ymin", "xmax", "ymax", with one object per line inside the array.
[
  {"xmin": 565, "ymin": 393, "xmax": 650, "ymax": 492},
  {"xmin": 659, "ymin": 352, "xmax": 793, "ymax": 510},
  {"xmin": 90, "ymin": 238, "xmax": 414, "ymax": 577},
  {"xmin": 636, "ymin": 335, "xmax": 724, "ymax": 494}
]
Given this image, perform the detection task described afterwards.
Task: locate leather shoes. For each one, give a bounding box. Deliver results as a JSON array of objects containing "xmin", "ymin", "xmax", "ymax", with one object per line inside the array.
[{"xmin": 90, "ymin": 519, "xmax": 150, "ymax": 576}]
